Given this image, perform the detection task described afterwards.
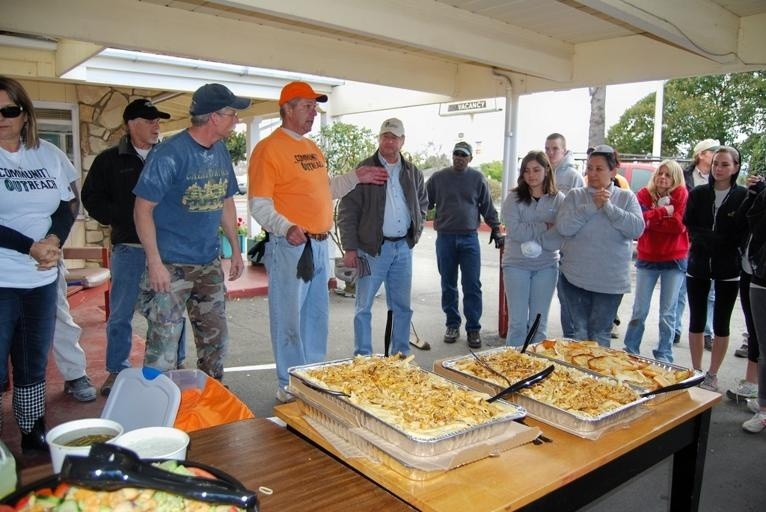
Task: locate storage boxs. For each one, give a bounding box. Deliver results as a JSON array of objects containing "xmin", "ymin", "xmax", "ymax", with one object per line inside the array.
[{"xmin": 97, "ymin": 367, "xmax": 255, "ymax": 436}]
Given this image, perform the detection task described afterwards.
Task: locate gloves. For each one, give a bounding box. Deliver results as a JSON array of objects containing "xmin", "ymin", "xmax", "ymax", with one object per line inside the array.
[{"xmin": 489, "ymin": 226, "xmax": 505, "ymax": 249}]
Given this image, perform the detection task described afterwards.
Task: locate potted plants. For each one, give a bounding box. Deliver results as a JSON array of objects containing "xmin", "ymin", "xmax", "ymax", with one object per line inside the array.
[{"xmin": 247, "ymin": 230, "xmax": 267, "ymax": 266}]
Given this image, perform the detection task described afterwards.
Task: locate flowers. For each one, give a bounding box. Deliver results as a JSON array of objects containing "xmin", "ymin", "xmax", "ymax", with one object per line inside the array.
[{"xmin": 219, "ymin": 217, "xmax": 248, "ymax": 238}]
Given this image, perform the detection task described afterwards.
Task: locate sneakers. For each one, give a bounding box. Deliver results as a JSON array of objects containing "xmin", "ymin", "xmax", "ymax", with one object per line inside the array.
[
  {"xmin": 19, "ymin": 434, "xmax": 51, "ymax": 458},
  {"xmin": 742, "ymin": 412, "xmax": 766, "ymax": 433},
  {"xmin": 274, "ymin": 387, "xmax": 295, "ymax": 403},
  {"xmin": 744, "ymin": 396, "xmax": 761, "ymax": 414},
  {"xmin": 466, "ymin": 330, "xmax": 482, "ymax": 348},
  {"xmin": 443, "ymin": 327, "xmax": 461, "ymax": 343},
  {"xmin": 734, "ymin": 339, "xmax": 749, "ymax": 358},
  {"xmin": 63, "ymin": 374, "xmax": 97, "ymax": 402},
  {"xmin": 100, "ymin": 372, "xmax": 119, "ymax": 396},
  {"xmin": 703, "ymin": 334, "xmax": 713, "ymax": 352},
  {"xmin": 698, "ymin": 371, "xmax": 720, "ymax": 392},
  {"xmin": 674, "ymin": 333, "xmax": 680, "ymax": 343},
  {"xmin": 726, "ymin": 377, "xmax": 760, "ymax": 405}
]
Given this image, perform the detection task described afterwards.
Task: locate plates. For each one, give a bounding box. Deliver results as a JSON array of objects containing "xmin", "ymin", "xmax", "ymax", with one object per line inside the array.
[{"xmin": 0, "ymin": 459, "xmax": 264, "ymax": 511}]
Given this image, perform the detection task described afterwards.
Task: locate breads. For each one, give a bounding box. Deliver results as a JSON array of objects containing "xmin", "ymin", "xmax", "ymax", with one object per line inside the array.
[{"xmin": 533, "ymin": 339, "xmax": 695, "ymax": 392}]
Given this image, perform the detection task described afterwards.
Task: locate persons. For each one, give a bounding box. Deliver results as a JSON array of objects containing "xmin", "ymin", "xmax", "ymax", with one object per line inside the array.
[
  {"xmin": 543, "ymin": 133, "xmax": 585, "ymax": 197},
  {"xmin": 336, "ymin": 119, "xmax": 429, "ymax": 362},
  {"xmin": 247, "ymin": 81, "xmax": 390, "ymax": 403},
  {"xmin": 0, "ymin": 76, "xmax": 76, "ymax": 456},
  {"xmin": 581, "ymin": 145, "xmax": 630, "ymax": 338},
  {"xmin": 742, "ymin": 185, "xmax": 764, "ymax": 433},
  {"xmin": 1, "ymin": 138, "xmax": 97, "ymax": 402},
  {"xmin": 499, "ymin": 152, "xmax": 565, "ymax": 349},
  {"xmin": 131, "ymin": 84, "xmax": 248, "ymax": 388},
  {"xmin": 733, "ymin": 173, "xmax": 764, "ymax": 358},
  {"xmin": 555, "ymin": 146, "xmax": 645, "ymax": 348},
  {"xmin": 423, "ymin": 140, "xmax": 502, "ymax": 349},
  {"xmin": 671, "ymin": 138, "xmax": 720, "ymax": 350},
  {"xmin": 79, "ymin": 98, "xmax": 186, "ymax": 399},
  {"xmin": 725, "ymin": 166, "xmax": 758, "ymax": 404},
  {"xmin": 623, "ymin": 161, "xmax": 687, "ymax": 364},
  {"xmin": 685, "ymin": 147, "xmax": 746, "ymax": 394}
]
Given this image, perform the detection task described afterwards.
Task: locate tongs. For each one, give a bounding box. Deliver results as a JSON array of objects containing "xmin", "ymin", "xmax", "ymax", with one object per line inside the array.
[{"xmin": 58, "ymin": 440, "xmax": 258, "ymax": 510}]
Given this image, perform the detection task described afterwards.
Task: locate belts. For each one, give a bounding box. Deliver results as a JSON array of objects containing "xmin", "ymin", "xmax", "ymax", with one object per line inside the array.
[
  {"xmin": 118, "ymin": 242, "xmax": 145, "ymax": 249},
  {"xmin": 307, "ymin": 234, "xmax": 329, "ymax": 241},
  {"xmin": 383, "ymin": 236, "xmax": 408, "ymax": 242}
]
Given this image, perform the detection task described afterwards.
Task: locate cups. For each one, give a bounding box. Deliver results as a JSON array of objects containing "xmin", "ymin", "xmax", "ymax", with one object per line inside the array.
[{"xmin": 44, "ymin": 418, "xmax": 189, "ymax": 473}]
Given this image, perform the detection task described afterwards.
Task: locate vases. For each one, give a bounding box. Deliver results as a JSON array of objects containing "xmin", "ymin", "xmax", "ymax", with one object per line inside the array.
[{"xmin": 219, "ymin": 233, "xmax": 245, "ymax": 260}]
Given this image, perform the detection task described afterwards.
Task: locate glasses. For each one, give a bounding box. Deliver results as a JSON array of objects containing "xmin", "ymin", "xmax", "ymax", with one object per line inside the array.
[
  {"xmin": 0, "ymin": 106, "xmax": 26, "ymax": 118},
  {"xmin": 453, "ymin": 150, "xmax": 469, "ymax": 158}
]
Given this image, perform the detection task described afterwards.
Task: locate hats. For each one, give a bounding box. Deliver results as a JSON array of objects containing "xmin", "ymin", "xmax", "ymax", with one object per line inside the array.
[
  {"xmin": 694, "ymin": 139, "xmax": 720, "ymax": 154},
  {"xmin": 453, "ymin": 141, "xmax": 474, "ymax": 158},
  {"xmin": 189, "ymin": 83, "xmax": 253, "ymax": 117},
  {"xmin": 378, "ymin": 118, "xmax": 405, "ymax": 137},
  {"xmin": 278, "ymin": 81, "xmax": 328, "ymax": 106},
  {"xmin": 122, "ymin": 98, "xmax": 171, "ymax": 122}
]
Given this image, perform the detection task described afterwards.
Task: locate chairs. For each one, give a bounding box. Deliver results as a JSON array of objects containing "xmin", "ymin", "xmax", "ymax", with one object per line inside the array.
[{"xmin": 61, "ymin": 246, "xmax": 110, "ymax": 323}]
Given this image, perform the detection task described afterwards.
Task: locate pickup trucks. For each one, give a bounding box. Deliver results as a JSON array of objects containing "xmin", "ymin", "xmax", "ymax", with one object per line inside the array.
[{"xmin": 616, "ymin": 162, "xmax": 661, "ymax": 197}]
